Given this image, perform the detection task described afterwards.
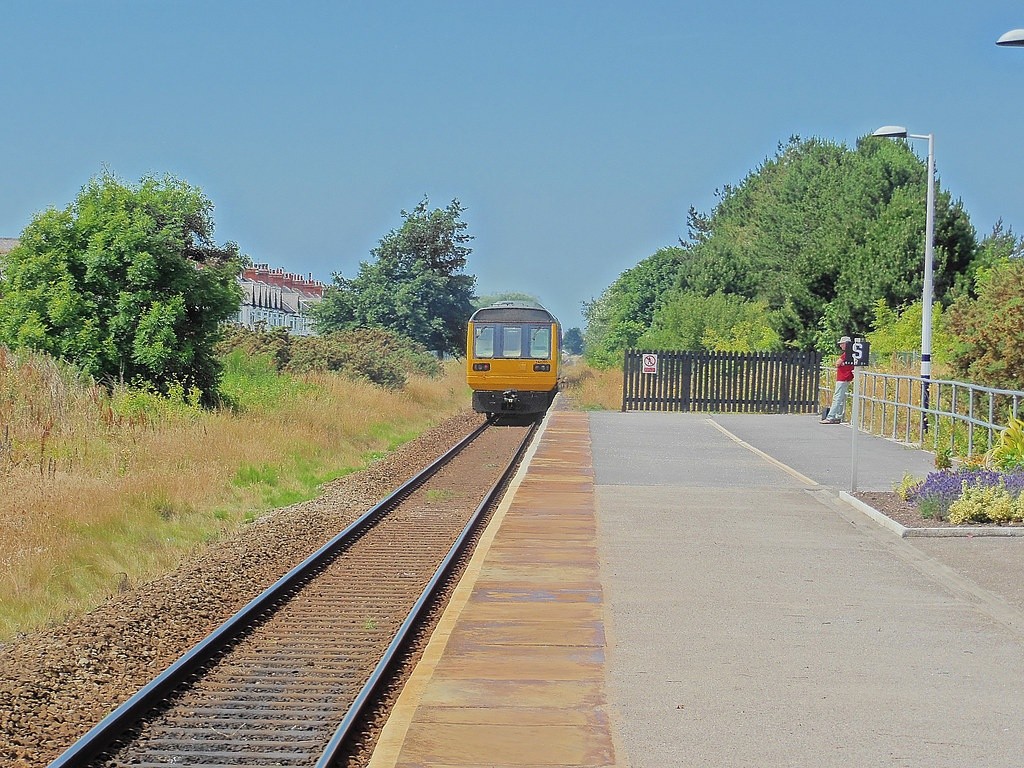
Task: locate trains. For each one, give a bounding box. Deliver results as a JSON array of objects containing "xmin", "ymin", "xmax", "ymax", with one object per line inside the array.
[{"xmin": 466, "ymin": 300, "xmax": 562, "ymax": 419}]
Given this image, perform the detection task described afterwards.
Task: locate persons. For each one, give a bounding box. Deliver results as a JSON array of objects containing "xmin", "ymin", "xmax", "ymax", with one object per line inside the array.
[{"xmin": 819, "ymin": 337, "xmax": 854, "ymax": 424}]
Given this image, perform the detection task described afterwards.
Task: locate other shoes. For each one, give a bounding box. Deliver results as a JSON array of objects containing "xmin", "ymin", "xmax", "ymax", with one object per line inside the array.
[{"xmin": 818, "ymin": 419, "xmax": 841, "ymax": 424}]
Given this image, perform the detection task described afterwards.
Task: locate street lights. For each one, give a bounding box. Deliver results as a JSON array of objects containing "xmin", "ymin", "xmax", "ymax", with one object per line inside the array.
[{"xmin": 871, "ymin": 126, "xmax": 934, "ymax": 433}]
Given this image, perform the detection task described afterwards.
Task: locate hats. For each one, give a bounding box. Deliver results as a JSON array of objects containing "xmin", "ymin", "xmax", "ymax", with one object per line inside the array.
[{"xmin": 837, "ymin": 336, "xmax": 851, "ymax": 343}]
